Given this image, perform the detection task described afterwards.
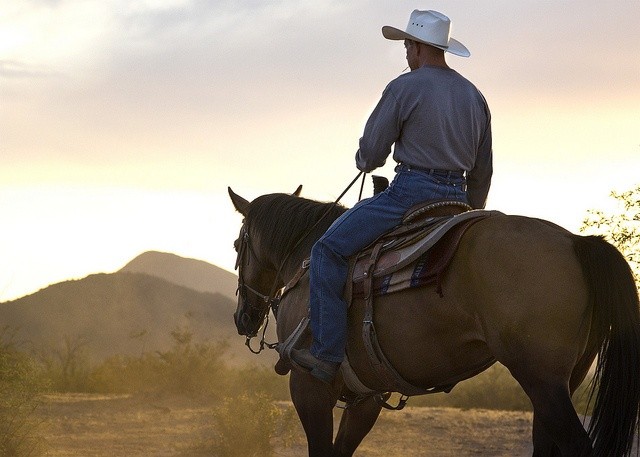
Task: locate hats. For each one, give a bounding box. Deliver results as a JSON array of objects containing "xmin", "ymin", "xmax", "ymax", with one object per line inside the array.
[{"xmin": 382, "ymin": 10, "xmax": 470, "ymax": 58}]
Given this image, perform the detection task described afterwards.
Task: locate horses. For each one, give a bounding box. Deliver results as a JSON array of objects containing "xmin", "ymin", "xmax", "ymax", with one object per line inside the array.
[{"xmin": 228, "ymin": 185, "xmax": 639, "ymax": 456}]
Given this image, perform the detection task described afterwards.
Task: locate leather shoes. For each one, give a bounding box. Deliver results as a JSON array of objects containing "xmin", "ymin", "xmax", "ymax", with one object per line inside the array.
[{"xmin": 274, "ymin": 343, "xmax": 338, "ymax": 389}]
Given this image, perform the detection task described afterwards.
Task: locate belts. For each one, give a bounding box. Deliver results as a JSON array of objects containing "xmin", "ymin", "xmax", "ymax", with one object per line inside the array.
[{"xmin": 395, "ymin": 162, "xmax": 465, "ymax": 184}]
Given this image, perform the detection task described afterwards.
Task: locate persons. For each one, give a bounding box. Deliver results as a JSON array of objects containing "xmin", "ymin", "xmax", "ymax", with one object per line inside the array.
[{"xmin": 287, "ymin": 8, "xmax": 493, "ymax": 386}]
